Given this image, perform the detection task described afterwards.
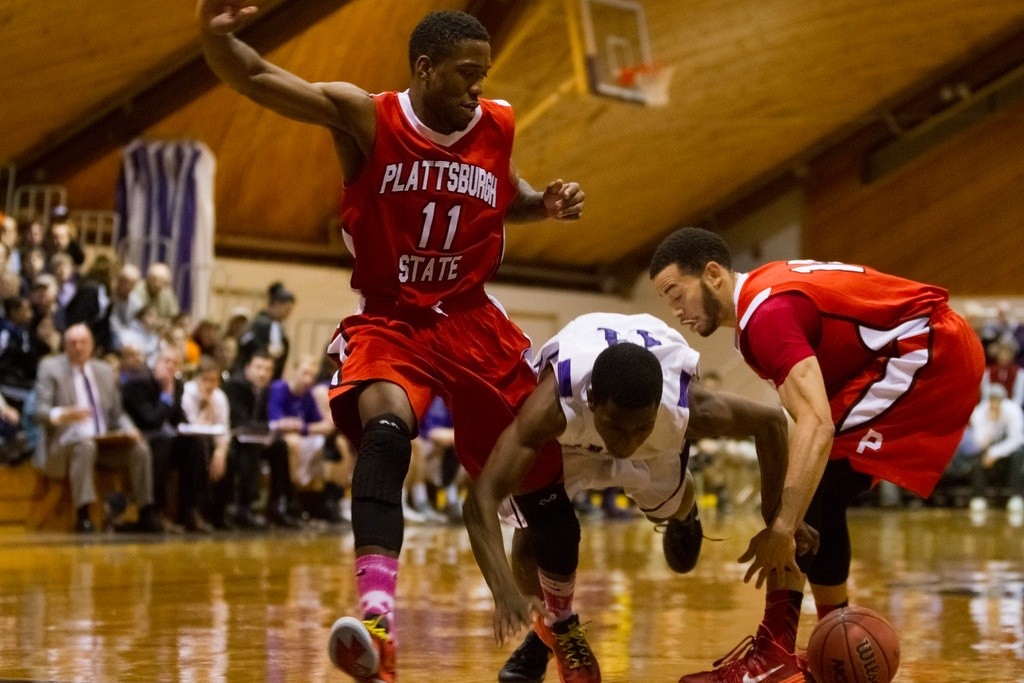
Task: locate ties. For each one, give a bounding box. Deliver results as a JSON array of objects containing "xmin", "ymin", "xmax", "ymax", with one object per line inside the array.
[{"xmin": 80, "ymin": 367, "xmax": 100, "ymax": 436}]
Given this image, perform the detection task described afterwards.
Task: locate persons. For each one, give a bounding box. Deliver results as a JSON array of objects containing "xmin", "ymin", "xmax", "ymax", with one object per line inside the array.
[
  {"xmin": 25, "ymin": 324, "xmax": 163, "ymax": 533},
  {"xmin": 0, "ymin": 207, "xmax": 251, "ymax": 468},
  {"xmin": 178, "ymin": 356, "xmax": 233, "ymax": 532},
  {"xmin": 646, "ymin": 223, "xmax": 985, "ymax": 683},
  {"xmin": 244, "ymin": 281, "xmax": 300, "ymax": 381},
  {"xmin": 122, "ymin": 353, "xmax": 210, "ymax": 534},
  {"xmin": 421, "ymin": 391, "xmax": 463, "ymax": 524},
  {"xmin": 459, "ymin": 309, "xmax": 826, "ymax": 681},
  {"xmin": 224, "ymin": 356, "xmax": 296, "ymax": 534},
  {"xmin": 266, "ymin": 353, "xmax": 340, "ymax": 527},
  {"xmin": 190, "ymin": 0, "xmax": 603, "ymax": 683},
  {"xmin": 398, "ymin": 436, "xmax": 448, "ymax": 526},
  {"xmin": 932, "ymin": 302, "xmax": 1023, "ymax": 510}
]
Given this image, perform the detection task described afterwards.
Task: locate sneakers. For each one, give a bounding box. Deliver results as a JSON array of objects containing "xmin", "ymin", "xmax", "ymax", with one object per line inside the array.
[
  {"xmin": 678, "ymin": 635, "xmax": 807, "ymax": 683},
  {"xmin": 661, "ymin": 501, "xmax": 703, "ymax": 574},
  {"xmin": 328, "ymin": 615, "xmax": 398, "ymax": 683},
  {"xmin": 497, "ymin": 630, "xmax": 554, "ymax": 683},
  {"xmin": 535, "ymin": 610, "xmax": 602, "ymax": 683}
]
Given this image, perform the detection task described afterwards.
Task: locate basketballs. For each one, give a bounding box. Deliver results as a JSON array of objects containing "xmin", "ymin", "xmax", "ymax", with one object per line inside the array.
[{"xmin": 806, "ymin": 606, "xmax": 901, "ymax": 683}]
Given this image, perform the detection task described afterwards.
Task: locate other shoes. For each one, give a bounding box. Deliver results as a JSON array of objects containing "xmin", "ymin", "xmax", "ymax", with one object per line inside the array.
[{"xmin": 68, "ymin": 486, "xmax": 645, "ymax": 535}]
